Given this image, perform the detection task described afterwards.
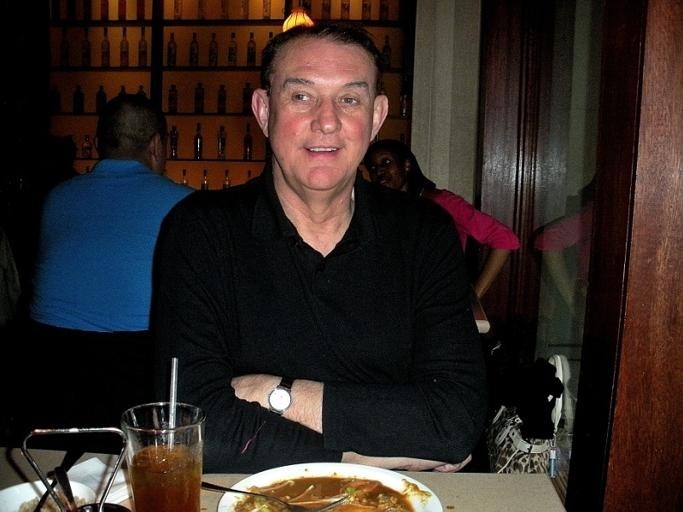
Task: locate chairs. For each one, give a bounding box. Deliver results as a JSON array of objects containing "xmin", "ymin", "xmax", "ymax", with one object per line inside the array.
[{"xmin": 1, "ymin": 311, "xmax": 155, "ymax": 456}]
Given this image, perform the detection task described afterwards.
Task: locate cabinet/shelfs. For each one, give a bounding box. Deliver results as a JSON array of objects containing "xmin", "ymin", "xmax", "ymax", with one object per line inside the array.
[{"xmin": 44, "ymin": 0, "xmax": 405, "ymax": 165}]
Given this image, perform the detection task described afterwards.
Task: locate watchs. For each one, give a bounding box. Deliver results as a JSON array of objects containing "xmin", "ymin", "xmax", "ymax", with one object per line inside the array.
[{"xmin": 268, "ymin": 375, "xmax": 295, "ymax": 414}]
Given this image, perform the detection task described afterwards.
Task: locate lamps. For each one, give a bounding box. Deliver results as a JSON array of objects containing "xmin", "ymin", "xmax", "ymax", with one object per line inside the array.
[{"xmin": 281, "ymin": 6, "xmax": 315, "ymax": 33}]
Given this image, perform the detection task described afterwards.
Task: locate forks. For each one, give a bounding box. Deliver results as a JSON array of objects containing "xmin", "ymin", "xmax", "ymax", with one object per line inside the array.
[{"xmin": 198, "ymin": 480, "xmax": 349, "ymax": 511}]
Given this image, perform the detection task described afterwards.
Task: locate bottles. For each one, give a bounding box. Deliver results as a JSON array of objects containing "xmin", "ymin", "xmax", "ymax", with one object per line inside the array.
[
  {"xmin": 166, "ymin": 0, "xmax": 274, "ymax": 190},
  {"xmin": 54, "ymin": 1, "xmax": 152, "ymax": 177},
  {"xmin": 300, "ymin": 0, "xmax": 408, "ymax": 146}
]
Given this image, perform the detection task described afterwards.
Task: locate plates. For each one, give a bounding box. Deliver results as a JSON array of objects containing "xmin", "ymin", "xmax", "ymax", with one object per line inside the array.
[
  {"xmin": 0, "ymin": 478, "xmax": 96, "ymax": 512},
  {"xmin": 217, "ymin": 462, "xmax": 444, "ymax": 511}
]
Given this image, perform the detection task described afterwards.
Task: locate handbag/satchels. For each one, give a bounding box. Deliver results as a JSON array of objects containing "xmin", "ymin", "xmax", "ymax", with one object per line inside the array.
[{"xmin": 487, "ymin": 353, "xmax": 564, "ymax": 473}]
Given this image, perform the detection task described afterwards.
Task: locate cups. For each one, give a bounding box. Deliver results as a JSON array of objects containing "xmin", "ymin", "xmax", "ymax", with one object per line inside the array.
[
  {"xmin": 75, "ymin": 501, "xmax": 131, "ymax": 512},
  {"xmin": 120, "ymin": 401, "xmax": 205, "ymax": 511}
]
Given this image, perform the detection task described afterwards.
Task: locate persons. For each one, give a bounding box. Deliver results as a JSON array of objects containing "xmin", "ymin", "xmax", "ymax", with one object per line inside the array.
[
  {"xmin": 365, "ymin": 141, "xmax": 519, "ymax": 298},
  {"xmin": 148, "ymin": 25, "xmax": 490, "ymax": 474},
  {"xmin": 535, "ymin": 204, "xmax": 596, "ymax": 321},
  {"xmin": 29, "ymin": 94, "xmax": 198, "ymax": 415}
]
